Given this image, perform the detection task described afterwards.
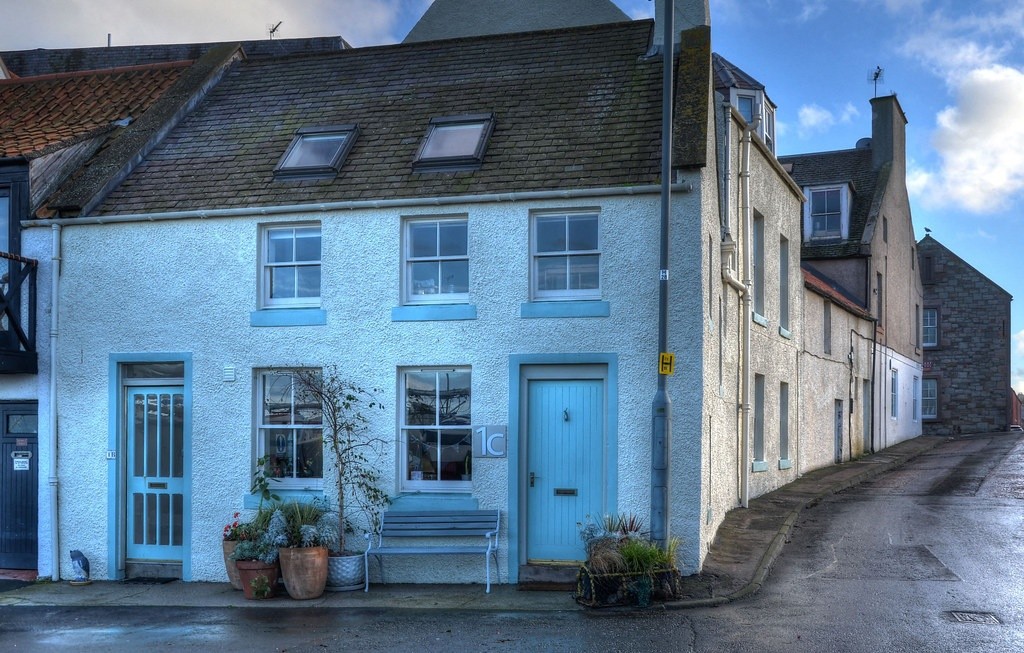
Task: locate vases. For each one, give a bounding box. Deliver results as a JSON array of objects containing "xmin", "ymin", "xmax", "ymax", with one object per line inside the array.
[{"xmin": 223, "ymin": 542, "xmax": 250, "ymax": 589}]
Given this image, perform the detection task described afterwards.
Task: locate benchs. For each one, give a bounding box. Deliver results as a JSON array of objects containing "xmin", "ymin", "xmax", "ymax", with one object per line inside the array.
[{"xmin": 365, "ymin": 509, "xmax": 502, "ymax": 594}]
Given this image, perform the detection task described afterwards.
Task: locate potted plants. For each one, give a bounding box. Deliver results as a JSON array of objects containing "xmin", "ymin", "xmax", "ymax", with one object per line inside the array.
[
  {"xmin": 462, "ymin": 451, "xmax": 471, "ymax": 481},
  {"xmin": 228, "ymin": 358, "xmax": 405, "ymax": 600},
  {"xmin": 577, "ymin": 510, "xmax": 681, "ymax": 611},
  {"xmin": 408, "ymin": 431, "xmax": 426, "ymax": 480}
]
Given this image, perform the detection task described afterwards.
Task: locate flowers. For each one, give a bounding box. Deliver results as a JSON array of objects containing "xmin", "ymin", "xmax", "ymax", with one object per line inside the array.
[{"xmin": 223, "ymin": 455, "xmax": 283, "ymax": 543}]
